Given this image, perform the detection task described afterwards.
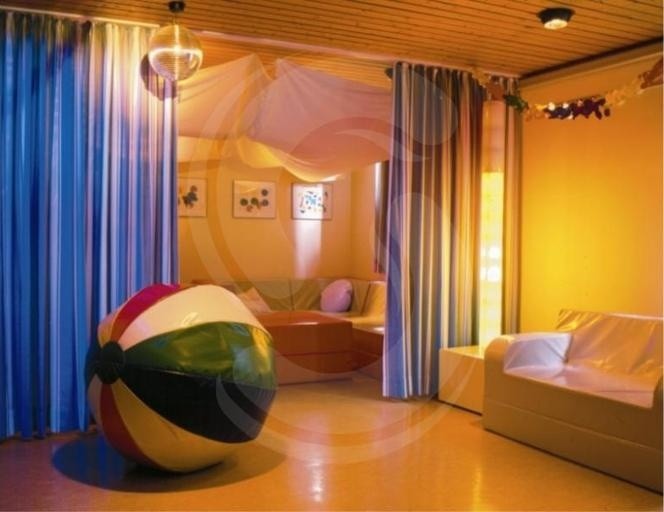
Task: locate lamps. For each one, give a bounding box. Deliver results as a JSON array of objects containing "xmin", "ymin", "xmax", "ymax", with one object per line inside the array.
[
  {"xmin": 537, "ymin": 7, "xmax": 576, "ymax": 31},
  {"xmin": 145, "ymin": 2, "xmax": 206, "ymax": 85}
]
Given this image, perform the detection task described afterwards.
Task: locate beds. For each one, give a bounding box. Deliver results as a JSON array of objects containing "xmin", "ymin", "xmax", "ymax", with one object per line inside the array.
[{"xmin": 437, "ymin": 343, "xmax": 490, "ymax": 416}]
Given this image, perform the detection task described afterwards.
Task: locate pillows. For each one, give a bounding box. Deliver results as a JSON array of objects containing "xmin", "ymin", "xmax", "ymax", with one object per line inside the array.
[
  {"xmin": 235, "ymin": 286, "xmax": 276, "ymax": 313},
  {"xmin": 319, "ymin": 278, "xmax": 354, "ymax": 313}
]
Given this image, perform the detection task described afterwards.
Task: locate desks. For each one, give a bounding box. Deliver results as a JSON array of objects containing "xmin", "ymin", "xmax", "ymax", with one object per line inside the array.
[{"xmin": 249, "ymin": 310, "xmax": 355, "ymax": 388}]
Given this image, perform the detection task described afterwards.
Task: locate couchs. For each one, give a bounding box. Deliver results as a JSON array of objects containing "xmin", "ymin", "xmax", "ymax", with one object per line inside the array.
[
  {"xmin": 481, "ymin": 305, "xmax": 663, "ymax": 496},
  {"xmin": 178, "ymin": 275, "xmax": 387, "ymax": 389}
]
